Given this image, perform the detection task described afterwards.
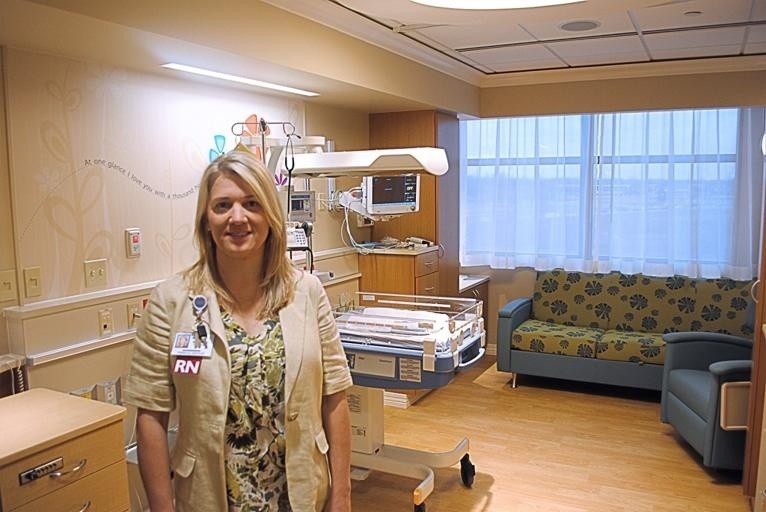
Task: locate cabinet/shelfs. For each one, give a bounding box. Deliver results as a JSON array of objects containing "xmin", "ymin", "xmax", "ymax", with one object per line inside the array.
[
  {"xmin": 358, "ymin": 109, "xmax": 490, "ymax": 404},
  {"xmin": 0, "ymin": 387, "xmax": 131, "ymax": 510}
]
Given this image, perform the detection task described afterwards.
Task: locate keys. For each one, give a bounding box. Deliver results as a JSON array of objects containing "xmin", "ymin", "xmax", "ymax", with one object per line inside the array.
[{"xmin": 192, "ymin": 317, "xmax": 208, "ymax": 349}]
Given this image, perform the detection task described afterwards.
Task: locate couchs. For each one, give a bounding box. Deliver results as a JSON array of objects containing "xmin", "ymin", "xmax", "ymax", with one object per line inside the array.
[{"xmin": 494, "ymin": 269, "xmax": 755, "ymax": 388}]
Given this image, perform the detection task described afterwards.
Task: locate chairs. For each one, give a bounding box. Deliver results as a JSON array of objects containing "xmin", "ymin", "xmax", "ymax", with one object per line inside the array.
[{"xmin": 661, "ymin": 330, "xmax": 754, "ymax": 473}]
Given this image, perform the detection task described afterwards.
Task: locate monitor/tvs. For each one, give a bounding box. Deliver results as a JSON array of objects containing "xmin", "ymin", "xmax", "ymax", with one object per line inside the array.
[{"xmin": 367, "ymin": 174, "xmax": 420, "ymax": 214}]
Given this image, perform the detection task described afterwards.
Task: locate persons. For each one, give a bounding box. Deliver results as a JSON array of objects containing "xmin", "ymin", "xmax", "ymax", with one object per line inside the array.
[{"xmin": 122, "ymin": 150, "xmax": 353, "ymax": 512}]
